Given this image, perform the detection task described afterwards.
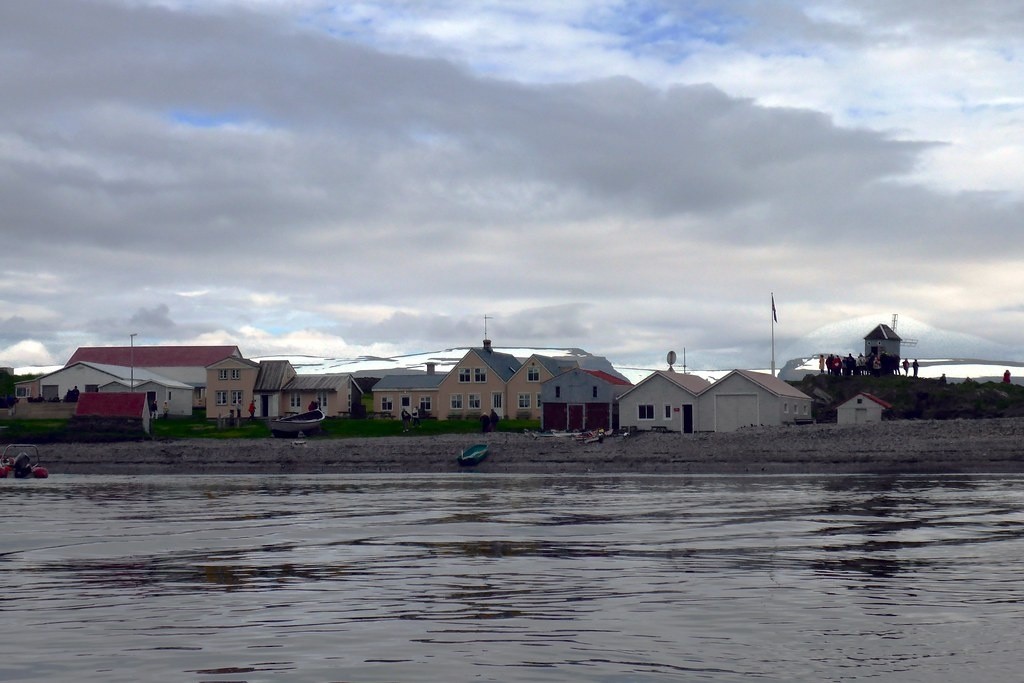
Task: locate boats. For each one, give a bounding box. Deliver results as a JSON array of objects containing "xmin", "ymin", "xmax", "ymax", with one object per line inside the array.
[
  {"xmin": 264, "ymin": 409, "xmax": 326, "ymax": 439},
  {"xmin": 456, "ymin": 442, "xmax": 490, "ymax": 467},
  {"xmin": 0, "ymin": 443, "xmax": 49, "ymax": 479},
  {"xmin": 524, "ymin": 425, "xmax": 616, "ymax": 444}
]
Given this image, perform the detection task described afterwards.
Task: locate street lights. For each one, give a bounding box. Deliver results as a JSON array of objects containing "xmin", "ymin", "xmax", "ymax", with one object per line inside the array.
[{"xmin": 130, "ymin": 333, "xmax": 139, "ymax": 392}]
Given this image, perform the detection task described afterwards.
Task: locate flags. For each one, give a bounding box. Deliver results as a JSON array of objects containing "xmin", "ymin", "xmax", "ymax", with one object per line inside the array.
[{"xmin": 772, "ymin": 295, "xmax": 777, "ymax": 322}]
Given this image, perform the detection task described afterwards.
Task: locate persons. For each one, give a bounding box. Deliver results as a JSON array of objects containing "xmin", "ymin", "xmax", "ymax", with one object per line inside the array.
[
  {"xmin": 490, "ymin": 408, "xmax": 499, "ymax": 432},
  {"xmin": 37, "ymin": 394, "xmax": 42, "ymax": 400},
  {"xmin": 308, "ymin": 401, "xmax": 318, "ymax": 411},
  {"xmin": 161, "ymin": 401, "xmax": 169, "ymax": 419},
  {"xmin": 401, "ymin": 407, "xmax": 409, "ymax": 432},
  {"xmin": 1002, "ymin": 370, "xmax": 1011, "ymax": 384},
  {"xmin": 70, "ymin": 385, "xmax": 80, "ymax": 403},
  {"xmin": 237, "ymin": 399, "xmax": 242, "ymax": 418},
  {"xmin": 412, "ymin": 406, "xmax": 422, "ymax": 428},
  {"xmin": 819, "ymin": 351, "xmax": 919, "ymax": 378},
  {"xmin": 248, "ymin": 400, "xmax": 256, "ymax": 421},
  {"xmin": 151, "ymin": 400, "xmax": 159, "ymax": 420},
  {"xmin": 53, "ymin": 394, "xmax": 59, "ymax": 401},
  {"xmin": 480, "ymin": 412, "xmax": 490, "ymax": 438},
  {"xmin": 7, "ymin": 395, "xmax": 12, "ymax": 407}
]
